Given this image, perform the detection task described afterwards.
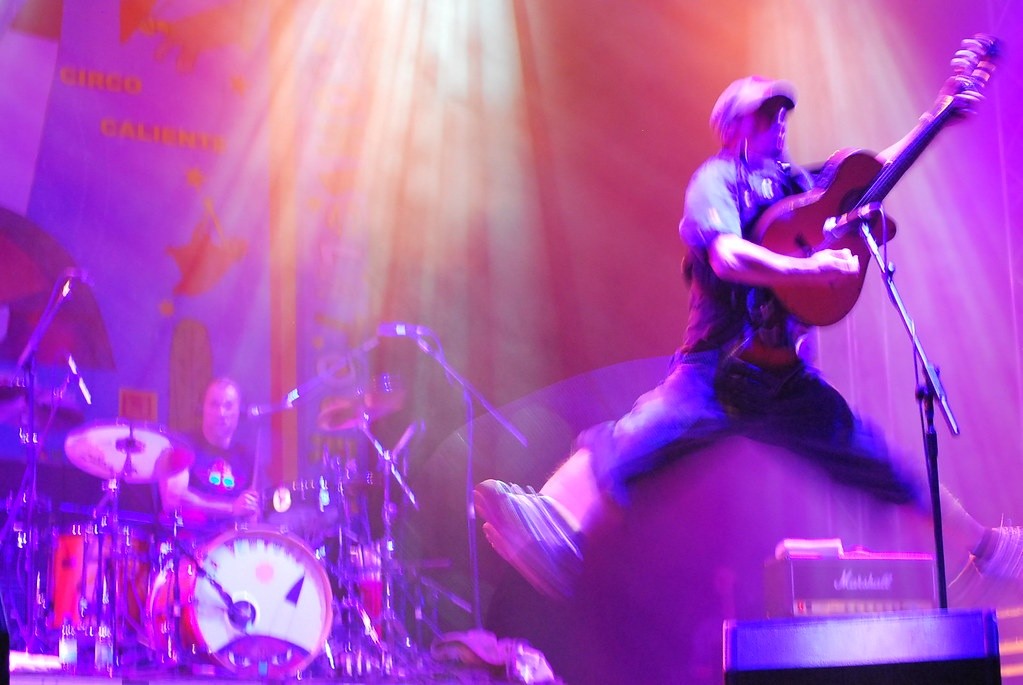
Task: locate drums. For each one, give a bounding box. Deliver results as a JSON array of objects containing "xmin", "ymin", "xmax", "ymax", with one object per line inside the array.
[
  {"xmin": 47, "ymin": 519, "xmax": 149, "ymax": 644},
  {"xmin": 139, "ymin": 525, "xmax": 334, "ymax": 678}
]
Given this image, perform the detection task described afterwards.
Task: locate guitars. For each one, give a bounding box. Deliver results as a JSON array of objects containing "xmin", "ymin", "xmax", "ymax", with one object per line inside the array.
[{"xmin": 749, "ymin": 28, "xmax": 1007, "ymax": 324}]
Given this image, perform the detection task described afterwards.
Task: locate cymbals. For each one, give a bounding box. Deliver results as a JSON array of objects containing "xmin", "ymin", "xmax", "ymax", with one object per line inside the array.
[
  {"xmin": 63, "ymin": 422, "xmax": 194, "ymax": 485},
  {"xmin": 311, "ymin": 381, "xmax": 408, "ymax": 430}
]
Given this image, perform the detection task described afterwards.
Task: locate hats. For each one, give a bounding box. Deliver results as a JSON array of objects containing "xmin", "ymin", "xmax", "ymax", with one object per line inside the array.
[{"xmin": 709, "ymin": 75, "xmax": 799, "ymax": 144}]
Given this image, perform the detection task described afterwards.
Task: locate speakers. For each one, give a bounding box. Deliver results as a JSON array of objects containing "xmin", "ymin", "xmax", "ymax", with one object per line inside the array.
[{"xmin": 722, "ymin": 556, "xmax": 1003, "ymax": 685}]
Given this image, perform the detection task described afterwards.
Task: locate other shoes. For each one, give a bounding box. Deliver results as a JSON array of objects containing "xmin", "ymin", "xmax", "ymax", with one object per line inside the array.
[
  {"xmin": 967, "ymin": 526, "xmax": 1023, "ymax": 581},
  {"xmin": 472, "ymin": 481, "xmax": 584, "ymax": 602}
]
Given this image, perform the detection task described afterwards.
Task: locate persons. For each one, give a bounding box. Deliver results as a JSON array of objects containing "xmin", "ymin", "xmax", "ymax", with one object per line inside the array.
[
  {"xmin": 472, "ymin": 73, "xmax": 1022, "ymax": 588},
  {"xmin": 159, "ymin": 371, "xmax": 268, "ymax": 528}
]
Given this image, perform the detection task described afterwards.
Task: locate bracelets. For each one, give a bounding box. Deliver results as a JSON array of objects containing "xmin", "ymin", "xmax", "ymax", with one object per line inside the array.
[{"xmin": 231, "ymin": 501, "xmax": 235, "ymax": 515}]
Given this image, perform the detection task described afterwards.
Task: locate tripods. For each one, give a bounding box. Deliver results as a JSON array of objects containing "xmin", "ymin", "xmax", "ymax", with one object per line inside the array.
[{"xmin": 319, "ymin": 405, "xmax": 453, "ymax": 678}]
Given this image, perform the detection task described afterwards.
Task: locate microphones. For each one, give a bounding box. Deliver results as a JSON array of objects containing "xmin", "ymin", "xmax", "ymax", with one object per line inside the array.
[
  {"xmin": 66, "ymin": 357, "xmax": 91, "ymax": 406},
  {"xmin": 823, "ymin": 203, "xmax": 882, "ymax": 242},
  {"xmin": 377, "ymin": 321, "xmax": 433, "ymax": 338},
  {"xmin": 247, "ymin": 401, "xmax": 297, "ymax": 419},
  {"xmin": 233, "ymin": 607, "xmax": 252, "ymax": 624}
]
